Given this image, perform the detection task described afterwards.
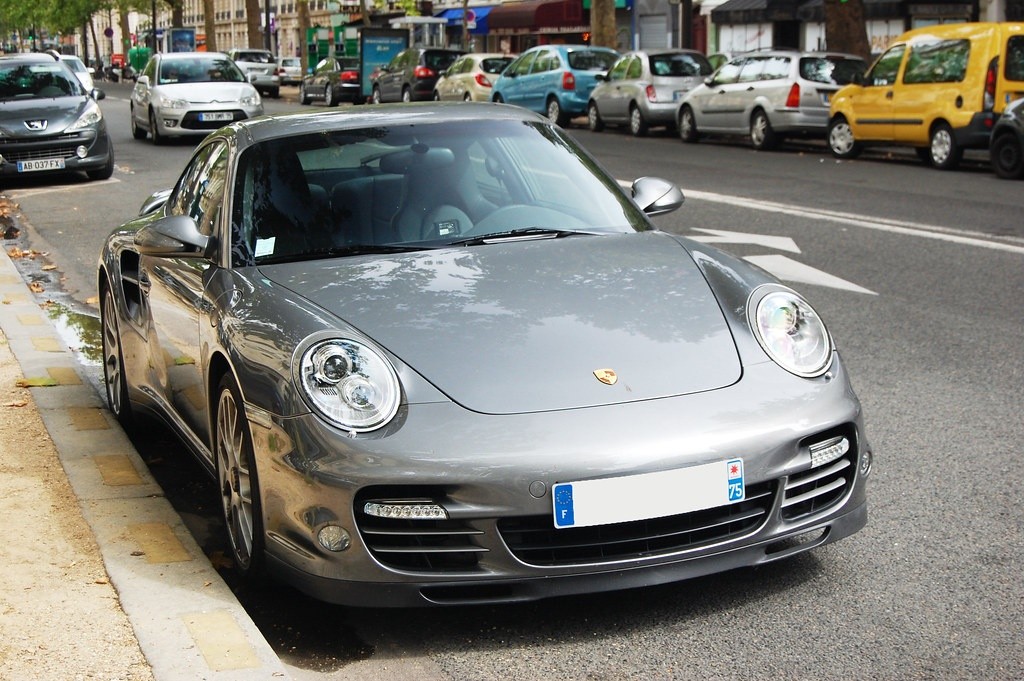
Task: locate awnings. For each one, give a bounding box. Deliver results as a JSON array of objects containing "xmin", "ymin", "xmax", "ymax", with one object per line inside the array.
[
  {"xmin": 340, "ymin": 12, "xmax": 405, "ymax": 27},
  {"xmin": 711, "ymin": 0, "xmax": 826, "ymax": 23},
  {"xmin": 440, "ymin": 7, "xmax": 489, "ymax": 34},
  {"xmin": 489, "ymin": 0, "xmax": 583, "ymax": 28}
]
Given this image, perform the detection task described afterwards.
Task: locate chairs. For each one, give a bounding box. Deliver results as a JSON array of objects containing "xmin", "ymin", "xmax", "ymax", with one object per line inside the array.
[
  {"xmin": 393, "ymin": 148, "xmax": 501, "ymax": 243},
  {"xmin": 248, "ymin": 152, "xmax": 334, "ymax": 258},
  {"xmin": 40, "ymin": 73, "xmax": 66, "ymax": 91}
]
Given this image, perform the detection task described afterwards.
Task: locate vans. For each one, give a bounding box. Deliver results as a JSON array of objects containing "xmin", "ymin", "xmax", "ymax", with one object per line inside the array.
[{"xmin": 826, "ymin": 22, "xmax": 1023, "ymax": 169}]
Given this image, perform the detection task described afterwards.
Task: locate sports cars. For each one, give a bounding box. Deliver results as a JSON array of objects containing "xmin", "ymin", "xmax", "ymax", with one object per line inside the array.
[{"xmin": 96, "ymin": 99, "xmax": 874, "ymax": 613}]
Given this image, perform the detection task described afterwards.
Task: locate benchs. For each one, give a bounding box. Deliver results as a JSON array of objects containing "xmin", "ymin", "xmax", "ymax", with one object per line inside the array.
[{"xmin": 308, "ymin": 172, "xmax": 402, "ymax": 244}]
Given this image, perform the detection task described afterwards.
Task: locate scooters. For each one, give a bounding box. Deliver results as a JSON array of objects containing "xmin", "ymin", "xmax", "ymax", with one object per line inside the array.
[{"xmin": 94, "ymin": 57, "xmax": 120, "ymax": 83}]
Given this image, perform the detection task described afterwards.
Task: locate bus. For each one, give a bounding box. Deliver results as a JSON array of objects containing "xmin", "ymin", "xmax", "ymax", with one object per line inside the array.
[
  {"xmin": 196, "ymin": 34, "xmax": 207, "ymax": 51},
  {"xmin": 142, "ymin": 29, "xmax": 165, "ymax": 50}
]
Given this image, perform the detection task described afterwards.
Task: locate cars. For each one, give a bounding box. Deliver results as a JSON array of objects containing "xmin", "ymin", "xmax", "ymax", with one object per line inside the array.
[
  {"xmin": 277, "ymin": 57, "xmax": 303, "ymax": 85},
  {"xmin": 3, "ymin": 44, "xmax": 17, "ymax": 54},
  {"xmin": 587, "ymin": 48, "xmax": 714, "ymax": 139},
  {"xmin": 488, "ymin": 45, "xmax": 623, "ymax": 128},
  {"xmin": 86, "ymin": 57, "xmax": 103, "ymax": 74},
  {"xmin": 60, "ymin": 56, "xmax": 95, "ymax": 97},
  {"xmin": 706, "ymin": 50, "xmax": 743, "ymax": 68},
  {"xmin": 987, "ymin": 95, "xmax": 1024, "ymax": 180},
  {"xmin": 431, "ymin": 53, "xmax": 521, "ymax": 101},
  {"xmin": 674, "ymin": 49, "xmax": 867, "ymax": 148},
  {"xmin": 129, "ymin": 51, "xmax": 264, "ymax": 147},
  {"xmin": 300, "ymin": 57, "xmax": 362, "ymax": 108},
  {"xmin": 372, "ymin": 46, "xmax": 469, "ymax": 103},
  {"xmin": 30, "ymin": 43, "xmax": 62, "ymax": 54}
]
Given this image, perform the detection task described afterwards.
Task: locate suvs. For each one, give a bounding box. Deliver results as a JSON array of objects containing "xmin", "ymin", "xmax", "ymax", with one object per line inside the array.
[
  {"xmin": 230, "ymin": 48, "xmax": 279, "ymax": 99},
  {"xmin": 0, "ymin": 50, "xmax": 115, "ymax": 183}
]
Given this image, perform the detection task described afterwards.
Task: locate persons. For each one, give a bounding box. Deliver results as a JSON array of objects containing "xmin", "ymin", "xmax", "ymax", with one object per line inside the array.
[
  {"xmin": 3, "ymin": 43, "xmax": 17, "ymax": 54},
  {"xmin": 109, "ymin": 61, "xmax": 137, "ymax": 82}
]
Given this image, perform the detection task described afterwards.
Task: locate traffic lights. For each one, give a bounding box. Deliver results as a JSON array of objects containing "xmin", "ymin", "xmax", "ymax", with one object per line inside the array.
[{"xmin": 29, "ymin": 29, "xmax": 33, "ymax": 36}]
